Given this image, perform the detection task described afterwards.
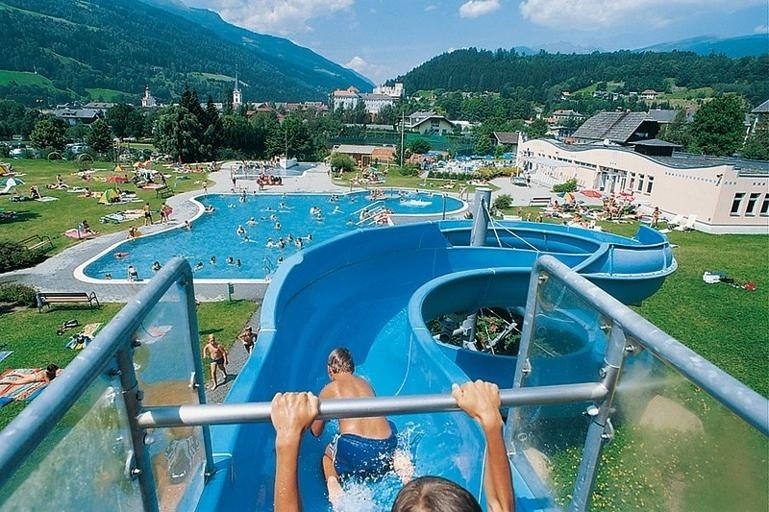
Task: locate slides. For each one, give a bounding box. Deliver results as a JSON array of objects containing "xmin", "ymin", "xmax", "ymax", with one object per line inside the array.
[{"xmin": 177, "ymin": 219, "xmax": 678, "ymax": 511}]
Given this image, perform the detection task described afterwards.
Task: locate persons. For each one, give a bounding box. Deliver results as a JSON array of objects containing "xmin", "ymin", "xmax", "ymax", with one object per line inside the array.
[
  {"xmin": 1, "ymin": 363, "xmax": 59, "ymax": 386},
  {"xmin": 1, "ymin": 154, "xmax": 421, "ymax": 284},
  {"xmin": 203, "ymin": 333, "xmax": 229, "ymax": 393},
  {"xmin": 270, "ymin": 379, "xmax": 516, "ymax": 512},
  {"xmin": 237, "ymin": 326, "xmax": 258, "ymax": 359},
  {"xmin": 440, "ymin": 162, "xmax": 664, "ymax": 231},
  {"xmin": 309, "ymin": 348, "xmax": 414, "ymax": 512}
]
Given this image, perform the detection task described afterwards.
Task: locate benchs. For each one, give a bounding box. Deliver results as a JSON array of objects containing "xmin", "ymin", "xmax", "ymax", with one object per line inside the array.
[
  {"xmin": 16, "ymin": 234, "xmax": 54, "ymax": 254},
  {"xmin": 35, "ymin": 292, "xmax": 100, "ymax": 313},
  {"xmin": 155, "ymin": 186, "xmax": 175, "ymax": 199}
]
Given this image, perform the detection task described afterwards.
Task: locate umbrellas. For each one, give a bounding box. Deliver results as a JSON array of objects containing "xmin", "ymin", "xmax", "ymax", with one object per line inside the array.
[{"xmin": 422, "ymin": 150, "xmax": 512, "ymax": 161}]
